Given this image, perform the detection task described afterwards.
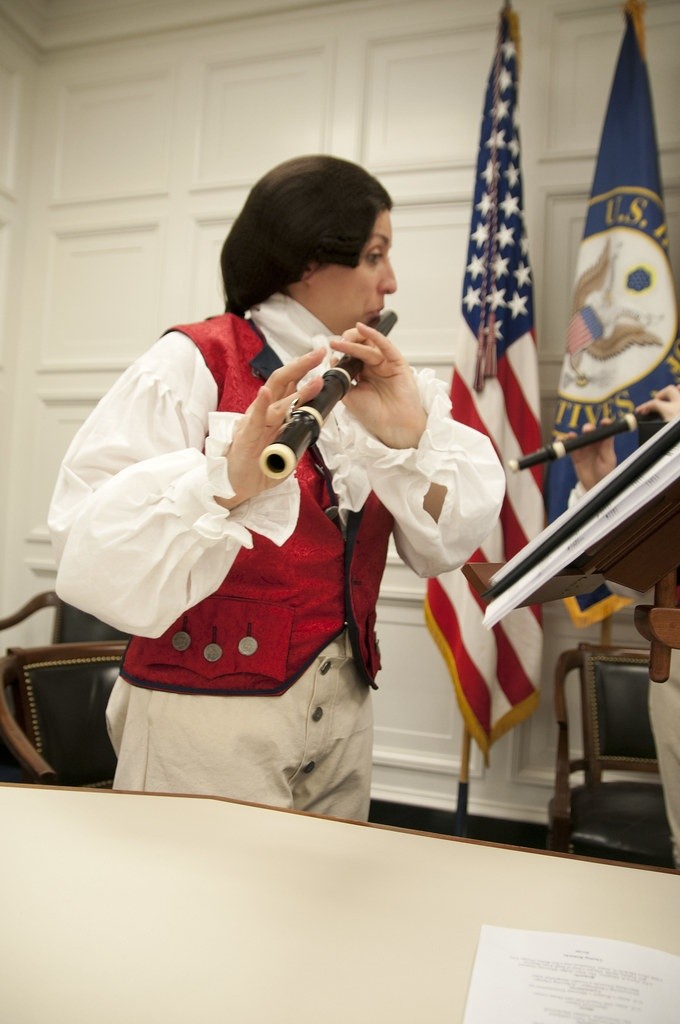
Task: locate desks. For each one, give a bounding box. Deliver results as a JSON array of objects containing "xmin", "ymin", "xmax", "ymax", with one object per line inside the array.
[{"xmin": 0, "ymin": 783, "xmax": 680, "ymax": 1024}]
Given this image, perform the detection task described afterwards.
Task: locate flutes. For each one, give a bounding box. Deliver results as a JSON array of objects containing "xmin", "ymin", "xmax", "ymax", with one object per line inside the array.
[
  {"xmin": 508, "ymin": 411, "xmax": 660, "ymax": 472},
  {"xmin": 259, "ymin": 310, "xmax": 399, "ymax": 480}
]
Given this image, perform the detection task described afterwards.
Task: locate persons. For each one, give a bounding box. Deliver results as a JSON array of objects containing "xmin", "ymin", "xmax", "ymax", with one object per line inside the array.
[
  {"xmin": 48, "ymin": 156, "xmax": 505, "ymax": 821},
  {"xmin": 566, "ymin": 383, "xmax": 680, "ymax": 869}
]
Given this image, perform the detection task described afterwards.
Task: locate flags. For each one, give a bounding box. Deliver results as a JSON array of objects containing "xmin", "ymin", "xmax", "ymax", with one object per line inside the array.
[
  {"xmin": 423, "ymin": 10, "xmax": 542, "ymax": 767},
  {"xmin": 548, "ymin": 8, "xmax": 680, "ymax": 627}
]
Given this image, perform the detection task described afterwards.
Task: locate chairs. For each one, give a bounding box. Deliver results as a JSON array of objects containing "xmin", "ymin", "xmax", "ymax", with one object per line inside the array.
[
  {"xmin": 548, "ymin": 641, "xmax": 673, "ymax": 869},
  {"xmin": 0, "ymin": 593, "xmax": 130, "ymax": 787}
]
